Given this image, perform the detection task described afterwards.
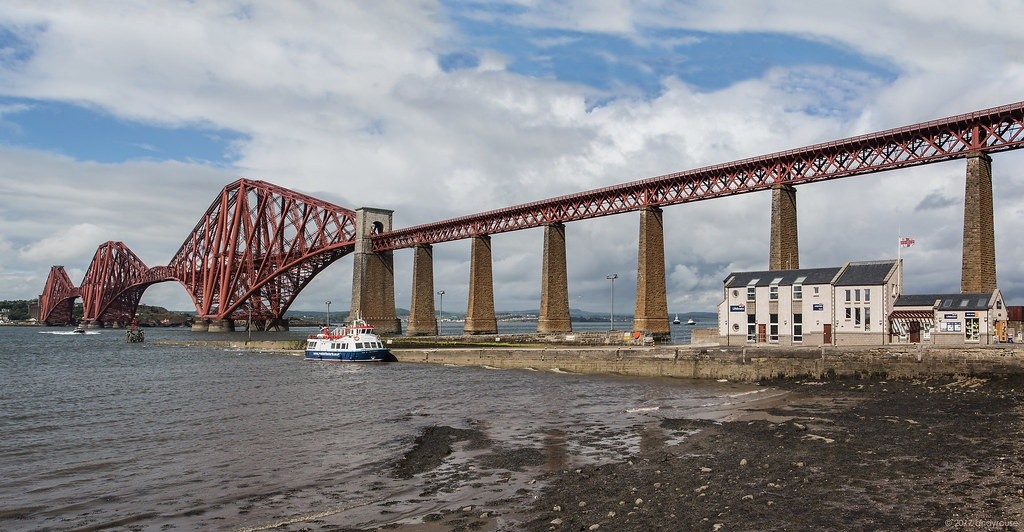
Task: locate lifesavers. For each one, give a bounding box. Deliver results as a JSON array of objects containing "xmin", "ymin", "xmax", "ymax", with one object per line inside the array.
[
  {"xmin": 377, "ymin": 336, "xmax": 380, "ymax": 340},
  {"xmin": 354, "ymin": 337, "xmax": 359, "ymax": 341}
]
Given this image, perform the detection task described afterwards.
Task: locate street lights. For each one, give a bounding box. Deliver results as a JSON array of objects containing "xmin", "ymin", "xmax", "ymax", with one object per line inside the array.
[
  {"xmin": 437, "ymin": 290, "xmax": 445, "ymax": 336},
  {"xmin": 606, "ymin": 273, "xmax": 618, "ymax": 332},
  {"xmin": 325, "ymin": 300, "xmax": 332, "ymax": 328}
]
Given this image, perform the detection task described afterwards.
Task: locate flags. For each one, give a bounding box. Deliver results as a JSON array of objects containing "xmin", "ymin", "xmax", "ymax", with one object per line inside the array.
[{"xmin": 898, "ymin": 237, "xmax": 916, "ymax": 248}]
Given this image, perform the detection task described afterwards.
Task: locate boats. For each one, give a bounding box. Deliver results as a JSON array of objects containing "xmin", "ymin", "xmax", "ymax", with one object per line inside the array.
[
  {"xmin": 687, "ymin": 316, "xmax": 696, "ymax": 325},
  {"xmin": 73, "ymin": 325, "xmax": 85, "ymax": 333},
  {"xmin": 673, "ymin": 312, "xmax": 681, "ymax": 324},
  {"xmin": 304, "ymin": 309, "xmax": 398, "ymax": 362}
]
